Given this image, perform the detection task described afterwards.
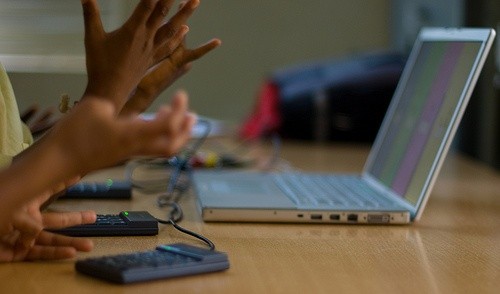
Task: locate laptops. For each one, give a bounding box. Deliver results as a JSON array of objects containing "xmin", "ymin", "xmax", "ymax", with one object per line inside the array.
[{"xmin": 188, "ymin": 25, "xmax": 497, "ymax": 223}]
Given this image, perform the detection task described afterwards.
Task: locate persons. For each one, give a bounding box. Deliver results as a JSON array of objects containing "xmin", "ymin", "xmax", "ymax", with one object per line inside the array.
[
  {"xmin": 19, "ymin": 101, "xmax": 58, "ymax": 134},
  {"xmin": 1, "ymin": 1, "xmax": 220, "ymax": 170},
  {"xmin": 1, "ymin": 91, "xmax": 196, "ymax": 262}
]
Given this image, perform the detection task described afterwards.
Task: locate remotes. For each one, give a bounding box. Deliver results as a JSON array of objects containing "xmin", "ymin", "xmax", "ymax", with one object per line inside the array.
[
  {"xmin": 42, "ymin": 211, "xmax": 160, "ymax": 237},
  {"xmin": 75, "ymin": 242, "xmax": 231, "ymax": 285},
  {"xmin": 57, "ymin": 180, "xmax": 131, "ymax": 200}
]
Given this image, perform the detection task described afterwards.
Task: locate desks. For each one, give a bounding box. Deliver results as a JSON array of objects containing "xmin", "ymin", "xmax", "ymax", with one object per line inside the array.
[{"xmin": 3, "ymin": 128, "xmax": 497, "ymax": 294}]
